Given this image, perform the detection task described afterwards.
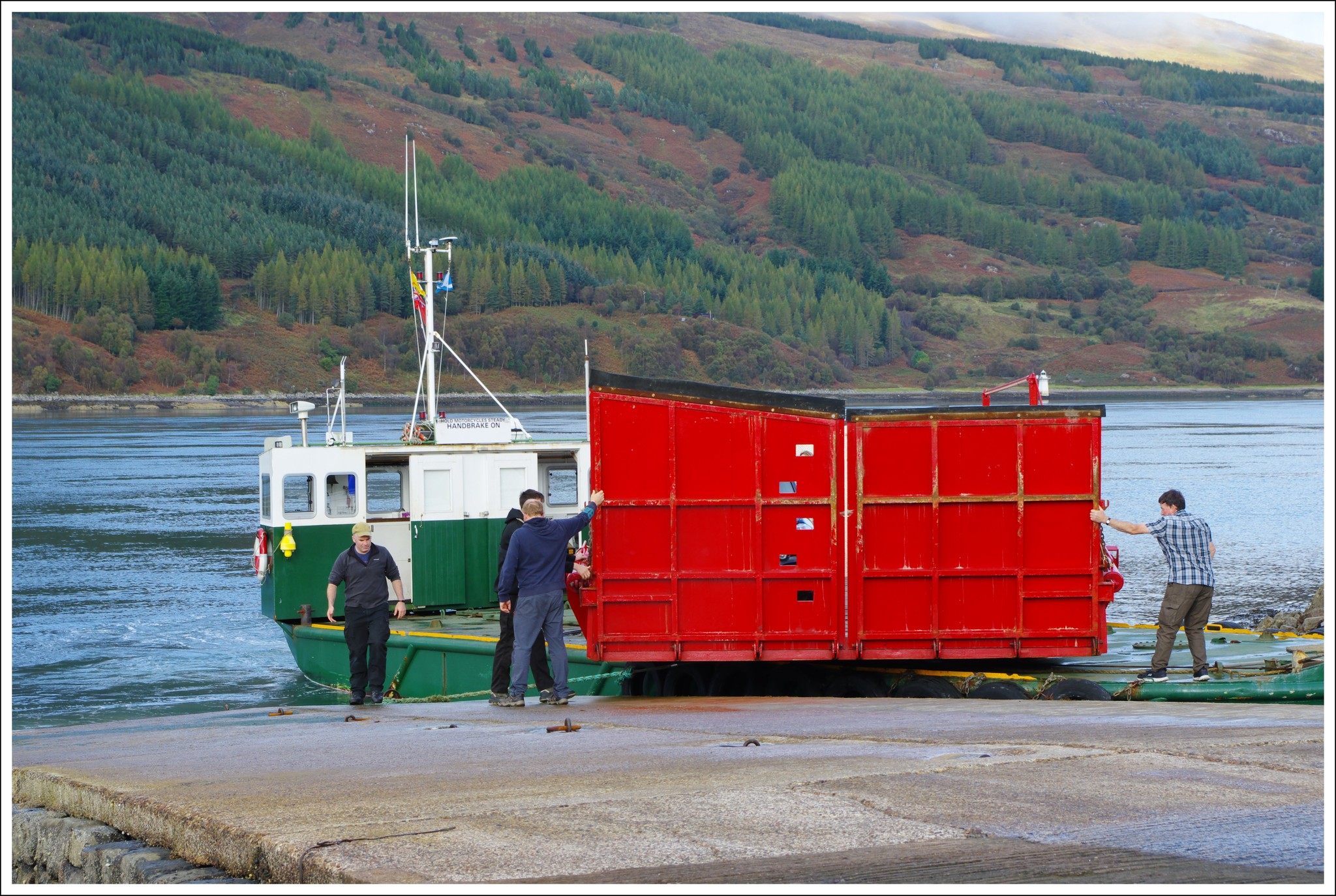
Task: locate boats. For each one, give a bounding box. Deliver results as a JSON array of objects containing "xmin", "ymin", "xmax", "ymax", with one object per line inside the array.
[{"xmin": 254, "ymin": 237, "xmax": 1324, "ymax": 702}]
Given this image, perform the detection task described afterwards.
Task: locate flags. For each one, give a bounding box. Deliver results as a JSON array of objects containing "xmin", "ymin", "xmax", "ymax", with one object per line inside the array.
[
  {"xmin": 435, "ymin": 272, "xmax": 453, "ymax": 294},
  {"xmin": 410, "ymin": 270, "xmax": 426, "ymax": 327}
]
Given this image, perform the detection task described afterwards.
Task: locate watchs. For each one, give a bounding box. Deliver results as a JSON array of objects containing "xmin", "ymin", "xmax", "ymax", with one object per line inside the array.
[{"xmin": 1106, "ymin": 518, "xmax": 1111, "ymax": 525}]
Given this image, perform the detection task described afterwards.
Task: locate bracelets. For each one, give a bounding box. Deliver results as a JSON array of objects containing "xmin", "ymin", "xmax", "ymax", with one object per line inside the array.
[{"xmin": 398, "ymin": 600, "xmax": 405, "ymax": 603}]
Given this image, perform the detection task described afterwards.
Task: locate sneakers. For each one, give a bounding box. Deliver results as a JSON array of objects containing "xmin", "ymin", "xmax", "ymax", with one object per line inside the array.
[
  {"xmin": 1192, "ymin": 666, "xmax": 1210, "ymax": 681},
  {"xmin": 540, "ymin": 687, "xmax": 576, "ymax": 703},
  {"xmin": 371, "ymin": 690, "xmax": 383, "ymax": 703},
  {"xmin": 489, "ymin": 692, "xmax": 510, "ymax": 705},
  {"xmin": 547, "ymin": 694, "xmax": 568, "ymax": 705},
  {"xmin": 498, "ymin": 693, "xmax": 525, "ymax": 707},
  {"xmin": 1137, "ymin": 667, "xmax": 1169, "ymax": 682},
  {"xmin": 349, "ymin": 693, "xmax": 364, "ymax": 705}
]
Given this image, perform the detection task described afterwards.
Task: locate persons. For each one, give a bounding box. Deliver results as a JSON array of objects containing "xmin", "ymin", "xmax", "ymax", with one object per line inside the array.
[
  {"xmin": 326, "ymin": 521, "xmax": 407, "ymax": 705},
  {"xmin": 488, "ymin": 488, "xmax": 605, "ymax": 708},
  {"xmin": 1089, "ymin": 489, "xmax": 1216, "ymax": 682}
]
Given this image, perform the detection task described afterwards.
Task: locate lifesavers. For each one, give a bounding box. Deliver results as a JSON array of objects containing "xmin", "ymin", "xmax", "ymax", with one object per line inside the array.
[{"xmin": 254, "ymin": 527, "xmax": 268, "ymax": 582}]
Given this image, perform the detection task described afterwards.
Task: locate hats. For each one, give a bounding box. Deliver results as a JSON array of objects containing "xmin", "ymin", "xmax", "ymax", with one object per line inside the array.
[{"xmin": 352, "ymin": 522, "xmax": 372, "ymax": 537}]
